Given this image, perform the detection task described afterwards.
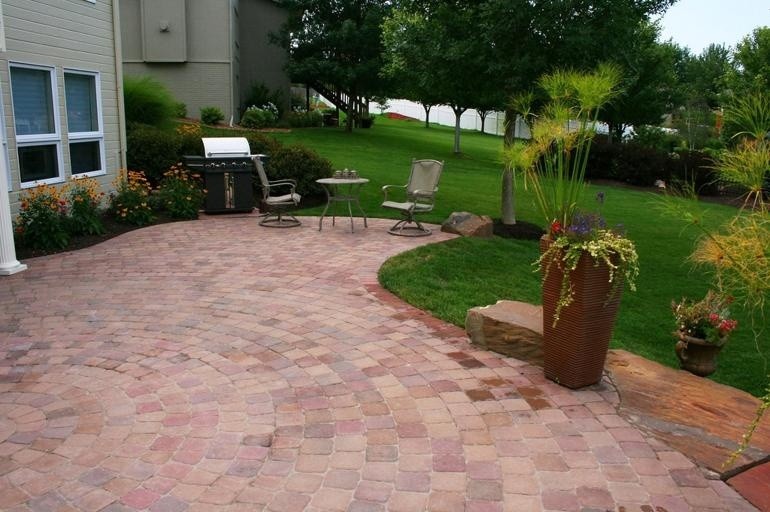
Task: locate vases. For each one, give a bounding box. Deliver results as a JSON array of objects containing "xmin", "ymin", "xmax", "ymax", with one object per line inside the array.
[{"xmin": 674, "ymin": 328, "xmax": 729, "ymax": 375}]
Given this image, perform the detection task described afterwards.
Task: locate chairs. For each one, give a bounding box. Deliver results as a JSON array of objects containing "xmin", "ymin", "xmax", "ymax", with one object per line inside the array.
[
  {"xmin": 251, "ymin": 155, "xmax": 305, "ymax": 228},
  {"xmin": 380, "ymin": 157, "xmax": 447, "ymax": 238}
]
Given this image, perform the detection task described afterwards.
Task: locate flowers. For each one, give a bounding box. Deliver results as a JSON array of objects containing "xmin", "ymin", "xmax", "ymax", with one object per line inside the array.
[
  {"xmin": 527, "ymin": 192, "xmax": 642, "ymax": 331},
  {"xmin": 669, "ymin": 290, "xmax": 740, "ymax": 337}
]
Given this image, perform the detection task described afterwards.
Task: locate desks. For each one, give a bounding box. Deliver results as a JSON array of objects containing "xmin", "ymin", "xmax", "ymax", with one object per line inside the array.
[{"xmin": 314, "ymin": 176, "xmax": 372, "ymax": 234}]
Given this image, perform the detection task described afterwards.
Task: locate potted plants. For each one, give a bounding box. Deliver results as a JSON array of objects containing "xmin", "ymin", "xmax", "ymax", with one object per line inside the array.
[{"xmin": 501, "ymin": 58, "xmax": 641, "ymax": 391}]
